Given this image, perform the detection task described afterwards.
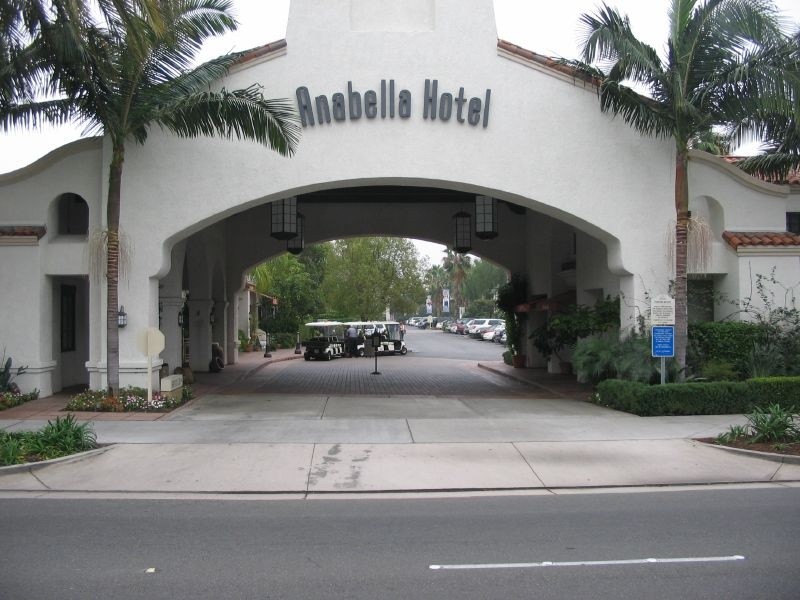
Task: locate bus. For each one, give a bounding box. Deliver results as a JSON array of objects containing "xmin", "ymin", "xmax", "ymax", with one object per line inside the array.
[{"xmin": 302, "ymin": 320, "xmax": 408, "ymax": 361}]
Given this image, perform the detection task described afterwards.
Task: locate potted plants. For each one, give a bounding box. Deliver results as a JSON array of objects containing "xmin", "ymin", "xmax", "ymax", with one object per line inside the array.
[
  {"xmin": 528, "ymin": 311, "xmax": 574, "ymax": 375},
  {"xmin": 495, "ymin": 274, "xmax": 526, "ymax": 367}
]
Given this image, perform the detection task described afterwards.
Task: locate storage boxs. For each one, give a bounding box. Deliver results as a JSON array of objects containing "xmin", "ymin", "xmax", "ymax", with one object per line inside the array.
[
  {"xmin": 161, "ymin": 373, "xmax": 183, "ymax": 391},
  {"xmin": 161, "ymin": 386, "xmax": 183, "ymax": 401}
]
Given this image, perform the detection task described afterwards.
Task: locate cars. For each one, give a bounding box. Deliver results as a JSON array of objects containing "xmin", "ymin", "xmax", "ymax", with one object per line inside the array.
[{"xmin": 405, "ymin": 316, "xmax": 508, "ymax": 344}]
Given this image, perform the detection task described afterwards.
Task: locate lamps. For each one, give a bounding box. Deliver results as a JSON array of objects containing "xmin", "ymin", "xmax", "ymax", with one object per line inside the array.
[
  {"xmin": 117, "ymin": 305, "xmax": 127, "ymax": 327},
  {"xmin": 286, "ymin": 212, "xmax": 305, "ymax": 255},
  {"xmin": 178, "ymin": 312, "xmax": 183, "ymax": 326},
  {"xmin": 271, "ymin": 197, "xmax": 298, "ymax": 241},
  {"xmin": 473, "ymin": 195, "xmax": 498, "ymax": 240},
  {"xmin": 452, "ymin": 211, "xmax": 472, "ymax": 253}
]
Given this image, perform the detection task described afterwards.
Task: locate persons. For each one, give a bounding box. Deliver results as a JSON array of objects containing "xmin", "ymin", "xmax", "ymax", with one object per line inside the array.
[
  {"xmin": 399, "ymin": 322, "xmax": 405, "ymax": 339},
  {"xmin": 346, "ymin": 325, "xmax": 357, "ymax": 358}
]
